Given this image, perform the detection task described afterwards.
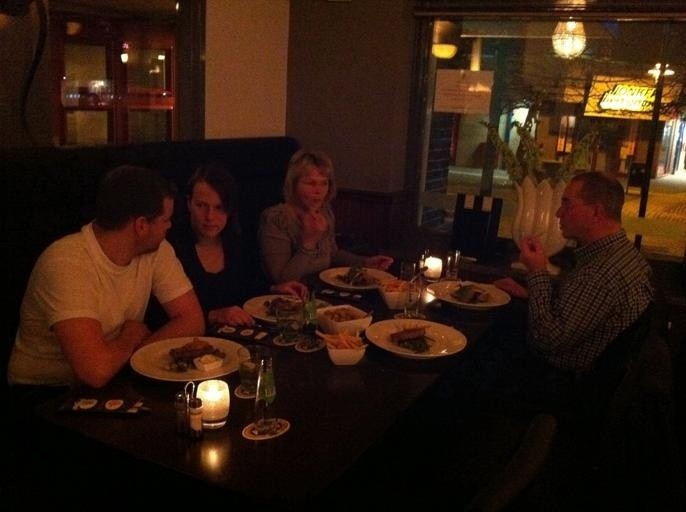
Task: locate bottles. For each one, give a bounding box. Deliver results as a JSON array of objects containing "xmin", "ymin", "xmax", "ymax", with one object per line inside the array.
[
  {"xmin": 188, "ymin": 397, "xmax": 204, "ymax": 436},
  {"xmin": 254, "ymin": 357, "xmax": 279, "ymax": 435},
  {"xmin": 300, "ymin": 289, "xmax": 318, "ymax": 346},
  {"xmin": 173, "ymin": 393, "xmax": 189, "ymax": 430}
]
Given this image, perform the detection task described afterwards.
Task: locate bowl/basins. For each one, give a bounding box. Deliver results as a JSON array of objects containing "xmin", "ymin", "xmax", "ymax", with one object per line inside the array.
[
  {"xmin": 319, "ymin": 303, "xmax": 372, "ymax": 335},
  {"xmin": 327, "ymin": 336, "xmax": 365, "ymax": 366},
  {"xmin": 378, "ymin": 282, "xmax": 418, "ymax": 310}
]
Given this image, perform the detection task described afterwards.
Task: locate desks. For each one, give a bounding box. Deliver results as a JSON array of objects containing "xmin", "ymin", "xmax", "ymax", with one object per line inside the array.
[{"xmin": 34, "ymin": 258, "xmax": 528, "ymax": 512}]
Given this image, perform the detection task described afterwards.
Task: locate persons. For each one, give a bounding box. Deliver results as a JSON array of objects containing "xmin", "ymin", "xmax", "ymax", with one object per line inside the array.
[
  {"xmin": 253, "ymin": 145, "xmax": 397, "ymax": 283},
  {"xmin": 495, "ymin": 168, "xmax": 656, "ymax": 379},
  {"xmin": 5, "ymin": 164, "xmax": 209, "ymax": 398},
  {"xmin": 166, "ymin": 167, "xmax": 314, "ymax": 331}
]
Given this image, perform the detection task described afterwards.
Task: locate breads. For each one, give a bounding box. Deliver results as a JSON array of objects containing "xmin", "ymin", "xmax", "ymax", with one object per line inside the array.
[
  {"xmin": 181, "ymin": 341, "xmax": 213, "ymax": 364},
  {"xmin": 391, "ymin": 328, "xmax": 425, "ymax": 343}
]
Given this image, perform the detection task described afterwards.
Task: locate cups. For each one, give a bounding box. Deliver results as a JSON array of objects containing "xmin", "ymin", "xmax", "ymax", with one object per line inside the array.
[
  {"xmin": 421, "ymin": 248, "xmax": 462, "ymax": 282},
  {"xmin": 238, "ymin": 343, "xmax": 271, "ymax": 393},
  {"xmin": 400, "ymin": 263, "xmax": 422, "ymax": 318},
  {"xmin": 198, "ymin": 380, "xmax": 231, "ymax": 429},
  {"xmin": 275, "ymin": 302, "xmax": 302, "ymax": 343}
]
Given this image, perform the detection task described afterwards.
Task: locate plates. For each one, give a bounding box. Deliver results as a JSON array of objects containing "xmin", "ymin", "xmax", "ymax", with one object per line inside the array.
[
  {"xmin": 426, "ymin": 281, "xmax": 512, "ymax": 308},
  {"xmin": 365, "ymin": 318, "xmax": 467, "ymax": 360},
  {"xmin": 243, "ymin": 294, "xmax": 331, "ymax": 324},
  {"xmin": 320, "ymin": 266, "xmax": 398, "ymax": 290},
  {"xmin": 130, "ymin": 337, "xmax": 249, "ymax": 381}
]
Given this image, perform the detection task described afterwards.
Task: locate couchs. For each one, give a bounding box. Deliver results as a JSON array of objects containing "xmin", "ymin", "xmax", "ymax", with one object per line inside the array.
[{"xmin": 0, "ymin": 137, "xmax": 302, "ymax": 416}]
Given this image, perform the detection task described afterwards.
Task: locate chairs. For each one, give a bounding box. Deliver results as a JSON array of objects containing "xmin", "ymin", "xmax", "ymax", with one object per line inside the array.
[
  {"xmin": 474, "ymin": 301, "xmax": 655, "ymax": 504},
  {"xmin": 466, "ymin": 414, "xmax": 558, "ymax": 512}
]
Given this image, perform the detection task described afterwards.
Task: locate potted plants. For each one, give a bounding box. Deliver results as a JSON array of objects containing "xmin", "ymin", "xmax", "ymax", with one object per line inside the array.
[{"xmin": 479, "ymin": 88, "xmax": 600, "ymax": 273}]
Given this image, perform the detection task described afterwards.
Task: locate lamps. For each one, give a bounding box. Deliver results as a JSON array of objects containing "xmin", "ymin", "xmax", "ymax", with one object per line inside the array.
[
  {"xmin": 552, "ymin": 21, "xmax": 587, "ymax": 60},
  {"xmin": 432, "ymin": 19, "xmax": 464, "ymax": 59}
]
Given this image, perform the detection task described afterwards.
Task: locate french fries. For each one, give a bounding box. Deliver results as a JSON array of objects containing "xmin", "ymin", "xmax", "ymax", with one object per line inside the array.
[
  {"xmin": 314, "ymin": 328, "xmax": 365, "ymax": 350},
  {"xmin": 378, "ymin": 280, "xmax": 418, "ymax": 292}
]
